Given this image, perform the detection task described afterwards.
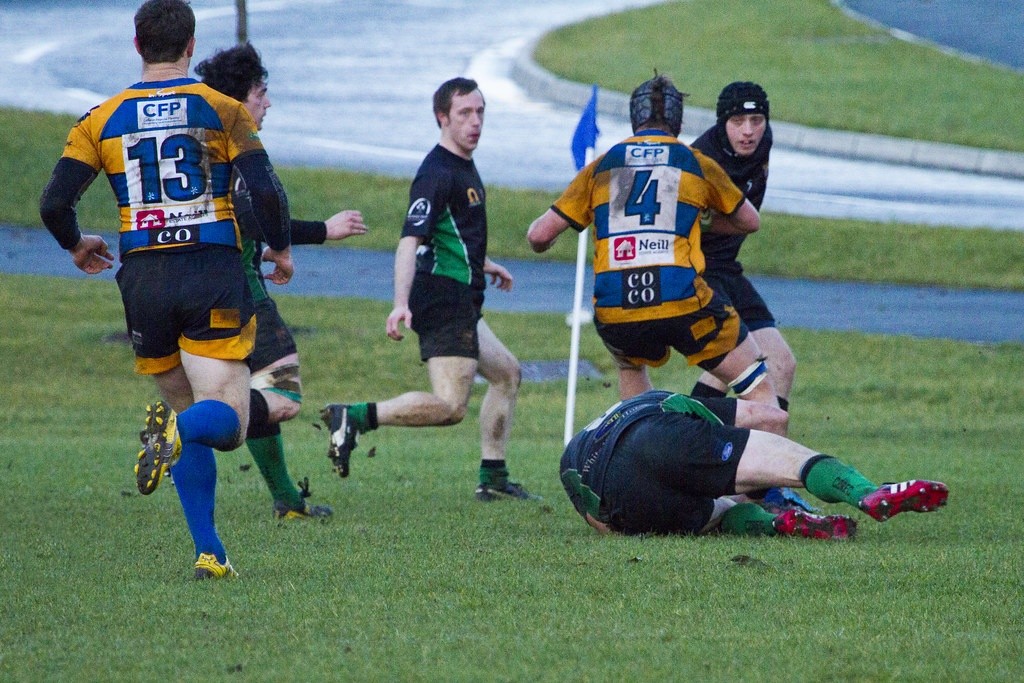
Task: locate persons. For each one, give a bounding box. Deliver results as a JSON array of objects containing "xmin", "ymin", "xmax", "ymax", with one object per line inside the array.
[
  {"xmin": 317, "ymin": 77, "xmax": 536, "ymax": 503},
  {"xmin": 681, "ymin": 80, "xmax": 799, "ymax": 413},
  {"xmin": 527, "ymin": 72, "xmax": 808, "ymax": 512},
  {"xmin": 188, "ymin": 39, "xmax": 369, "ymax": 521},
  {"xmin": 559, "ymin": 388, "xmax": 949, "ymax": 540},
  {"xmin": 39, "ymin": 0, "xmax": 294, "ymax": 581}
]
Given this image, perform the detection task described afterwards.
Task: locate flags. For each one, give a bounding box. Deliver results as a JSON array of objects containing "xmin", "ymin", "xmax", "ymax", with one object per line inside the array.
[{"xmin": 570, "ymin": 84, "xmax": 600, "ymax": 169}]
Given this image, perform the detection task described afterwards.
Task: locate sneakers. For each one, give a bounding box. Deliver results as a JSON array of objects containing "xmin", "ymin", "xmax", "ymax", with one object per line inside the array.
[
  {"xmin": 476, "ymin": 482, "xmax": 542, "ymax": 504},
  {"xmin": 133, "ymin": 401, "xmax": 182, "ymax": 495},
  {"xmin": 194, "ymin": 553, "xmax": 239, "ymax": 580},
  {"xmin": 272, "ymin": 496, "xmax": 332, "ymax": 526},
  {"xmin": 320, "ymin": 403, "xmax": 360, "ymax": 478},
  {"xmin": 772, "ymin": 509, "xmax": 856, "ymax": 539},
  {"xmin": 859, "ymin": 479, "xmax": 949, "ymax": 522}
]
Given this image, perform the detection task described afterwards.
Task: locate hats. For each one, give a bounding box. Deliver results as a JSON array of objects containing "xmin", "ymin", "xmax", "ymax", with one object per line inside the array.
[
  {"xmin": 630, "ymin": 77, "xmax": 683, "ymax": 138},
  {"xmin": 716, "ymin": 81, "xmax": 769, "ymax": 131}
]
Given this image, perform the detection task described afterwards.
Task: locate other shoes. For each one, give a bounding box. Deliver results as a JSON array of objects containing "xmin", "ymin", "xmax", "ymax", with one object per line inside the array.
[{"xmin": 762, "ymin": 486, "xmax": 814, "ymax": 513}]
